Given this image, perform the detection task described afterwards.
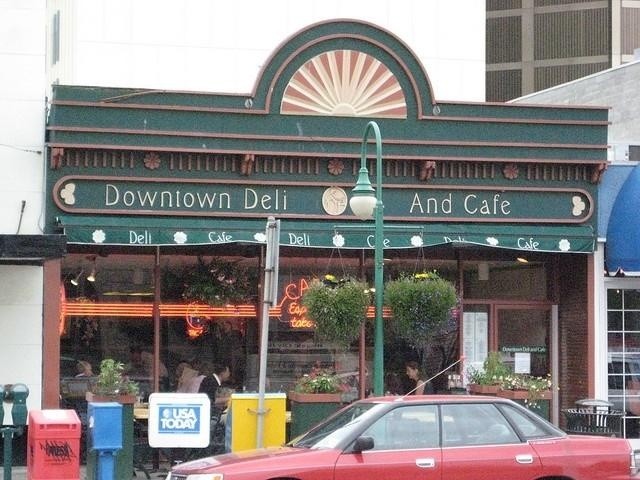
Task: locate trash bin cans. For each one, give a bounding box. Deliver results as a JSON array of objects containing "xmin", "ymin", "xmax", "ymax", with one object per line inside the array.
[{"xmin": 561, "ymin": 408, "xmax": 628, "ymax": 438}]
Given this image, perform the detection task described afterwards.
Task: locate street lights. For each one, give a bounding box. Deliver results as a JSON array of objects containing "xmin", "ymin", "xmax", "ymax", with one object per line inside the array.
[{"xmin": 349, "ymin": 117, "xmax": 389, "ymax": 396}]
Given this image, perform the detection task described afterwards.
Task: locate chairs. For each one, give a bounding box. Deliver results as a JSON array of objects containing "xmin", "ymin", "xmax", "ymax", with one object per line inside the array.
[{"xmin": 133, "ymin": 367, "xmax": 226, "ymax": 475}]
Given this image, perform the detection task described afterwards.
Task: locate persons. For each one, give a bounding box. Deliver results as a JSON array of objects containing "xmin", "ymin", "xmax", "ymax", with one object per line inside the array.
[
  {"xmin": 403, "ymin": 361, "xmax": 433, "ymax": 395},
  {"xmin": 71, "ymin": 345, "xmax": 230, "ymax": 472},
  {"xmin": 348, "ymin": 366, "xmax": 370, "ymax": 390}
]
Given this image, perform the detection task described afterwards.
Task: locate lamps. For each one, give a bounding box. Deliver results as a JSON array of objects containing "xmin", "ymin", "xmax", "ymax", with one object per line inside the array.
[{"xmin": 71, "ymin": 266, "xmax": 100, "ymax": 287}]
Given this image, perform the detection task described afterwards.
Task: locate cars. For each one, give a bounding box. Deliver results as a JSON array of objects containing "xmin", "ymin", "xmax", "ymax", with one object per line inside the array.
[
  {"xmin": 311, "ymin": 370, "xmax": 359, "ymax": 392},
  {"xmin": 167, "ymin": 350, "xmax": 638, "ymax": 480},
  {"xmin": 59, "ymin": 355, "xmax": 101, "ymax": 391}
]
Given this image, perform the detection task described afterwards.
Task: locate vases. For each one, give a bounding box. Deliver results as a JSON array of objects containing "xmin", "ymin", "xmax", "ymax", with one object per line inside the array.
[
  {"xmin": 320, "ymin": 279, "xmax": 351, "ymax": 290},
  {"xmin": 498, "ymin": 387, "xmax": 553, "ymax": 424},
  {"xmin": 414, "ymin": 278, "xmax": 439, "ymax": 284}
]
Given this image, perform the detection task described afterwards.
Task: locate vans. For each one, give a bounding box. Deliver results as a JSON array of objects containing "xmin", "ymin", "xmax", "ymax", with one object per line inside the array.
[{"xmin": 605, "ymin": 343, "xmax": 639, "ymax": 418}]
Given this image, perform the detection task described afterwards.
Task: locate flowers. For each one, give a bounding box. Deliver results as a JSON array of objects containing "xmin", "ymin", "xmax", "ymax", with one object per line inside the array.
[
  {"xmin": 302, "ymin": 273, "xmax": 370, "ymax": 356},
  {"xmin": 491, "ymin": 374, "xmax": 561, "ymax": 409},
  {"xmin": 384, "ymin": 270, "xmax": 459, "ymax": 353},
  {"xmin": 183, "ymin": 259, "xmax": 258, "ymax": 308}
]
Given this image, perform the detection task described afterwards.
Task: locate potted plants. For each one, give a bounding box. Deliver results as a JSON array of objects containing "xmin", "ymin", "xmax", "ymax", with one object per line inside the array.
[
  {"xmin": 289, "ymin": 367, "xmax": 354, "ymax": 448},
  {"xmin": 84, "ymin": 358, "xmax": 140, "ymax": 480},
  {"xmin": 467, "ymin": 350, "xmax": 511, "ymax": 395}
]
